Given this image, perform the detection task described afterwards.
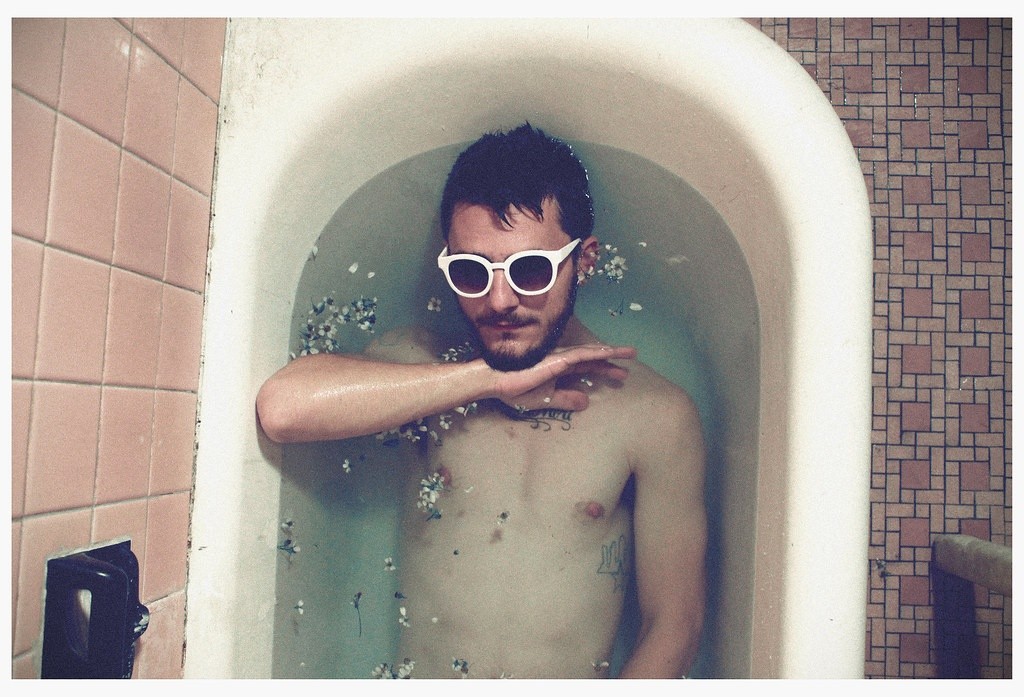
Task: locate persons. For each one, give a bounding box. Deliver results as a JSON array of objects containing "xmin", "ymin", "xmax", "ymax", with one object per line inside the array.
[{"xmin": 257, "ymin": 121, "xmax": 706, "ymax": 679}]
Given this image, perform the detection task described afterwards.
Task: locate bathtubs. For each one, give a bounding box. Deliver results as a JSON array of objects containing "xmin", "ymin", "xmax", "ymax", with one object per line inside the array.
[{"xmin": 180, "ymin": 17, "xmax": 872, "ymax": 679}]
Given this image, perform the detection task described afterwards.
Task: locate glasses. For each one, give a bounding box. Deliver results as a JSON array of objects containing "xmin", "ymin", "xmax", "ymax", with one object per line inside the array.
[{"xmin": 437, "ymin": 237, "xmax": 583, "ymax": 298}]
